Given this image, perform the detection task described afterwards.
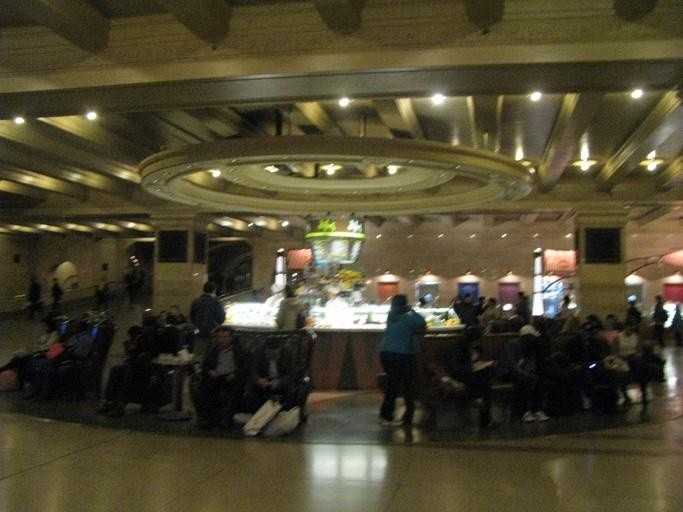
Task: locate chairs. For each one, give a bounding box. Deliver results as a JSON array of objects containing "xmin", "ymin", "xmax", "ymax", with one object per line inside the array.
[
  {"xmin": 196, "ymin": 329, "xmax": 314, "ymax": 421},
  {"xmin": 103, "ymin": 314, "xmax": 194, "ymax": 421},
  {"xmin": 30, "ymin": 316, "xmax": 116, "ymax": 400}
]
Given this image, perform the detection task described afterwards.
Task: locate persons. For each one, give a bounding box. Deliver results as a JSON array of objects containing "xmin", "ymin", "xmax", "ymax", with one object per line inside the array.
[
  {"xmin": 126, "ymin": 268, "xmax": 138, "ymax": 305},
  {"xmin": 483, "ymin": 297, "xmax": 496, "ymax": 311},
  {"xmin": 587, "ymin": 313, "xmax": 603, "ymax": 328},
  {"xmin": 653, "ymin": 294, "xmax": 669, "ymax": 347},
  {"xmin": 453, "ymin": 295, "xmax": 475, "ymax": 324},
  {"xmin": 95, "ymin": 277, "xmax": 116, "ymax": 312},
  {"xmin": 27, "ymin": 277, "xmax": 44, "ymax": 320},
  {"xmin": 189, "ymin": 280, "xmax": 227, "ymax": 327},
  {"xmin": 278, "ymin": 285, "xmax": 307, "ymax": 333},
  {"xmin": 569, "ymin": 324, "xmax": 608, "ymax": 415},
  {"xmin": 171, "ymin": 304, "xmax": 188, "ymax": 325},
  {"xmin": 22, "ymin": 318, "xmax": 83, "ymax": 401},
  {"xmin": 56, "ymin": 318, "xmax": 109, "ymax": 403},
  {"xmin": 606, "ymin": 313, "xmax": 622, "ymax": 330},
  {"xmin": 198, "ymin": 326, "xmax": 246, "ymax": 433},
  {"xmin": 515, "ymin": 291, "xmax": 528, "ymax": 319},
  {"xmin": 561, "ymin": 296, "xmax": 570, "ymax": 308},
  {"xmin": 627, "ymin": 300, "xmax": 641, "ymax": 331},
  {"xmin": 250, "ymin": 334, "xmax": 304, "ymax": 422},
  {"xmin": 378, "ymin": 293, "xmax": 427, "ymax": 427},
  {"xmin": 447, "ymin": 329, "xmax": 503, "ymax": 433},
  {"xmin": 475, "ymin": 296, "xmax": 486, "ymax": 316},
  {"xmin": 400, "ymin": 326, "xmax": 426, "ymax": 425},
  {"xmin": 464, "ymin": 294, "xmax": 473, "ymax": 303},
  {"xmin": 50, "ymin": 278, "xmax": 64, "ymax": 311},
  {"xmin": 509, "ymin": 324, "xmax": 553, "ymax": 423},
  {"xmin": 609, "ymin": 321, "xmax": 649, "ymax": 408},
  {"xmin": 96, "ymin": 326, "xmax": 160, "ymax": 416}
]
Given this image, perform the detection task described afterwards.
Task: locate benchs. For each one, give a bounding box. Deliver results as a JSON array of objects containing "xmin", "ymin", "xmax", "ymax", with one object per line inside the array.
[{"xmin": 422, "ymin": 331, "xmax": 642, "ymax": 432}]
